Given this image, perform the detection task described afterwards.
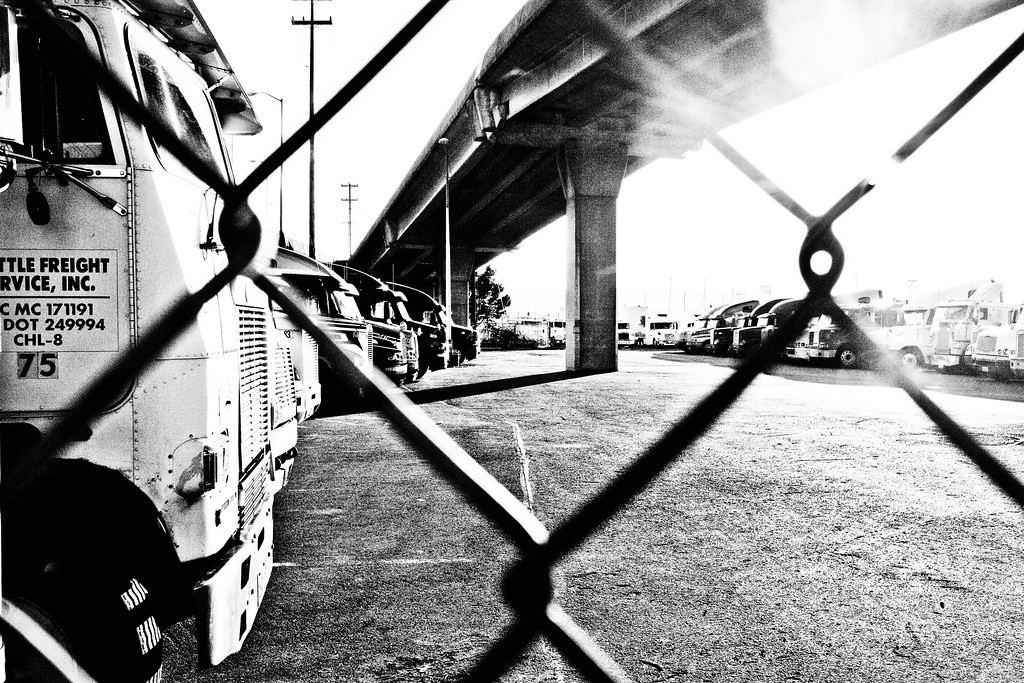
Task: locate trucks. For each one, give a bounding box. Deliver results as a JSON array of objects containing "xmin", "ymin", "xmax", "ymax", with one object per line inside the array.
[
  {"xmin": 515, "ymin": 317, "xmax": 550, "ymax": 350},
  {"xmin": 869, "ymin": 305, "xmax": 928, "ymax": 374},
  {"xmin": 973, "ymin": 304, "xmax": 1024, "ymax": 381},
  {"xmin": 781, "ymin": 290, "xmax": 909, "ymax": 369},
  {"xmin": 384, "ymin": 282, "xmax": 454, "ymax": 372},
  {"xmin": 277, "ymin": 246, "xmax": 380, "ymax": 405},
  {"xmin": 678, "ymin": 296, "xmax": 818, "ymax": 357},
  {"xmin": 451, "ymin": 317, "xmax": 482, "ymax": 368},
  {"xmin": 546, "ymin": 319, "xmax": 569, "ymax": 347},
  {"xmin": 326, "ymin": 262, "xmax": 407, "ymax": 391},
  {"xmin": 927, "ymin": 281, "xmax": 1003, "ymax": 375},
  {"xmin": 0, "ymin": 0, "xmax": 299, "ymax": 683},
  {"xmin": 634, "ymin": 314, "xmax": 681, "ymax": 348},
  {"xmin": 617, "ymin": 322, "xmax": 636, "ymax": 348}
]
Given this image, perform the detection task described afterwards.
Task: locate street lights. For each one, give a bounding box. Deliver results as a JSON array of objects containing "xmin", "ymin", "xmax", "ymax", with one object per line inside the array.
[{"xmin": 438, "ymin": 138, "xmax": 455, "ymax": 368}]
